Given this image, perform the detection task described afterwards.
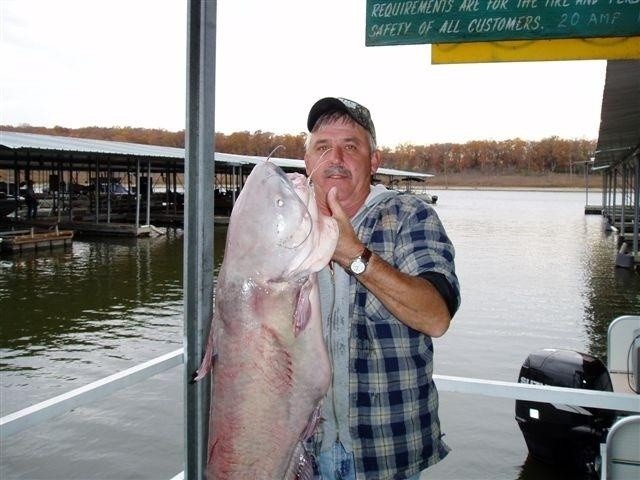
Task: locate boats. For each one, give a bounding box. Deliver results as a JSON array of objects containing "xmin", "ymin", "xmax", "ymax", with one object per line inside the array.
[
  {"xmin": 515, "ymin": 314, "xmax": 640, "ymax": 480},
  {"xmin": 0, "ymin": 226, "xmax": 73, "ymax": 253}
]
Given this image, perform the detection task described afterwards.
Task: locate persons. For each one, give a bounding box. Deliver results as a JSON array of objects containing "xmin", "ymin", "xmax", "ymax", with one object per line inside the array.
[
  {"xmin": 301, "ymin": 93, "xmax": 462, "ymax": 479},
  {"xmin": 25, "ymin": 188, "xmax": 41, "ymax": 222}
]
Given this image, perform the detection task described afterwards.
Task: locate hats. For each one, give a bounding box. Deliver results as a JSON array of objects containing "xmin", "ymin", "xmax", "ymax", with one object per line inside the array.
[{"xmin": 306, "ymin": 96, "xmax": 377, "ymax": 146}]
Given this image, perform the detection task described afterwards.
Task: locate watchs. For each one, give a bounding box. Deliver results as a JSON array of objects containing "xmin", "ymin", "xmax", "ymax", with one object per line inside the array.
[{"xmin": 344, "ymin": 246, "xmax": 373, "ymax": 281}]
